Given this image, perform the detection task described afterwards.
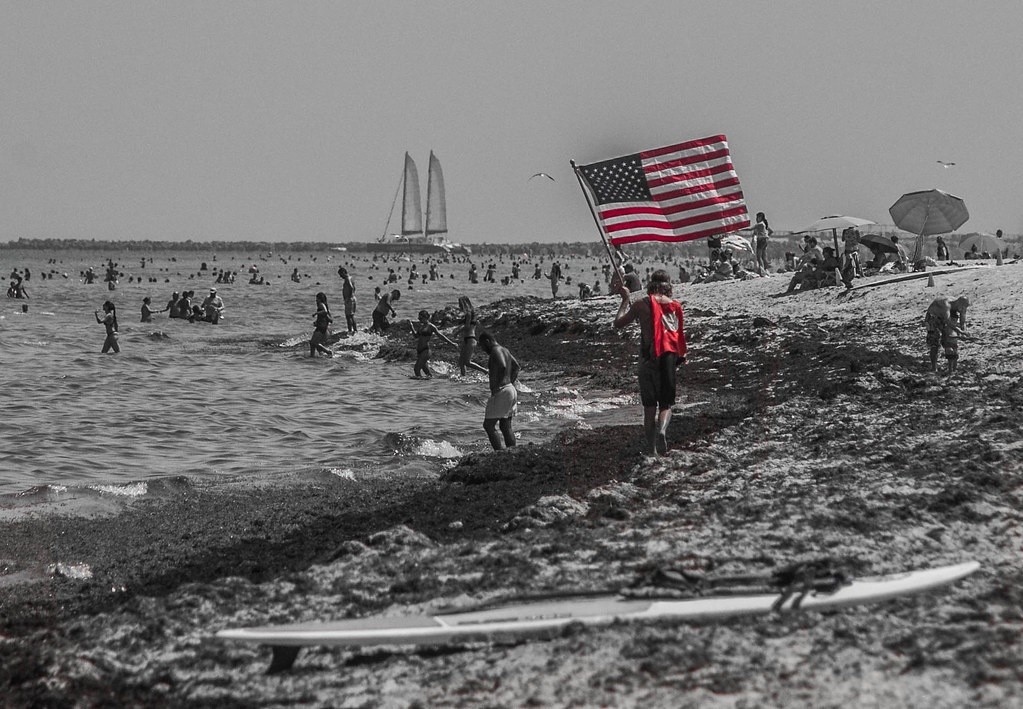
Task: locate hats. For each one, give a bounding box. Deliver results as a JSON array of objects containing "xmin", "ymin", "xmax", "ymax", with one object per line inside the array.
[
  {"xmin": 209, "ymin": 288, "xmax": 218, "ymax": 294},
  {"xmin": 623, "ymin": 264, "xmax": 634, "ymax": 272},
  {"xmin": 143, "ymin": 296, "xmax": 150, "ymax": 303}
]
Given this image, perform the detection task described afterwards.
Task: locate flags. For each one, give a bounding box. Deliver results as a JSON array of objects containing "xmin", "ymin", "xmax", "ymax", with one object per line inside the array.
[{"xmin": 578, "ymin": 134, "xmax": 750, "ymax": 244}]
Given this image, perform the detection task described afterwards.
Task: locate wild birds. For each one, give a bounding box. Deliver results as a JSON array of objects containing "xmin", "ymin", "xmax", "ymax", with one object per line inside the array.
[
  {"xmin": 937, "ymin": 160, "xmax": 956, "ymax": 167},
  {"xmin": 526, "ymin": 172, "xmax": 556, "ymax": 182}
]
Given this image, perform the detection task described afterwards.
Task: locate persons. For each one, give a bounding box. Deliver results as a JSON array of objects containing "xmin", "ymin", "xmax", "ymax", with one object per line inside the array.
[
  {"xmin": 925, "ymin": 296, "xmax": 971, "ymax": 373},
  {"xmin": 784, "ymin": 235, "xmax": 838, "ymax": 294},
  {"xmin": 693, "ymin": 234, "xmax": 741, "ymax": 284},
  {"xmin": 452, "ymin": 296, "xmax": 489, "ymax": 375},
  {"xmin": 866, "ymin": 236, "xmax": 907, "ymax": 268},
  {"xmin": 480, "ymin": 332, "xmax": 520, "ymax": 452},
  {"xmin": 7, "ymin": 248, "xmax": 612, "ymax": 358},
  {"xmin": 737, "ymin": 212, "xmax": 773, "ymax": 270},
  {"xmin": 615, "ymin": 244, "xmax": 707, "ymax": 293},
  {"xmin": 842, "ymin": 227, "xmax": 864, "ymax": 277},
  {"xmin": 937, "ymin": 236, "xmax": 949, "ymax": 261},
  {"xmin": 965, "ymin": 246, "xmax": 989, "ymax": 259},
  {"xmin": 616, "ymin": 270, "xmax": 686, "ymax": 456},
  {"xmin": 407, "ymin": 311, "xmax": 458, "ymax": 379},
  {"xmin": 996, "ymin": 230, "xmax": 1003, "ymax": 239}
]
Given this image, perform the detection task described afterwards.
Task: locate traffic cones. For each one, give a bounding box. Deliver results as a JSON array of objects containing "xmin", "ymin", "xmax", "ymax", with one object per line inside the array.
[
  {"xmin": 996, "ymin": 246, "xmax": 1005, "ymax": 266},
  {"xmin": 927, "ymin": 272, "xmax": 934, "ymax": 287}
]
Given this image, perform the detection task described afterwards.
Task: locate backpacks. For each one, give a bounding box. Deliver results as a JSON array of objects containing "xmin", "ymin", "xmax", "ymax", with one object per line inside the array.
[{"xmin": 913, "ymin": 260, "xmax": 926, "ymax": 272}]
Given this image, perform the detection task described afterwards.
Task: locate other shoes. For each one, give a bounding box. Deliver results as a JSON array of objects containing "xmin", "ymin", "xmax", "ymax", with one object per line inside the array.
[
  {"xmin": 618, "ymin": 569, "xmax": 695, "ymax": 600},
  {"xmin": 765, "ymin": 563, "xmax": 836, "ymax": 591}
]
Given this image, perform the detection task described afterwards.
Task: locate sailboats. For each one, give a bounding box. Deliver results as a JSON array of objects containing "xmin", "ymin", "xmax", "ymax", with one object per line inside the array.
[{"xmin": 366, "ymin": 148, "xmax": 473, "ymax": 255}]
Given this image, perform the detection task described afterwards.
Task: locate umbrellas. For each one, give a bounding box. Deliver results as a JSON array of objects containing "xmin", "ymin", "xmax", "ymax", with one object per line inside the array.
[
  {"xmin": 859, "ymin": 234, "xmax": 899, "ymax": 252},
  {"xmin": 959, "ymin": 234, "xmax": 1005, "ymax": 253},
  {"xmin": 801, "ymin": 214, "xmax": 878, "ymax": 257},
  {"xmin": 889, "ymin": 188, "xmax": 970, "ymax": 251}
]
